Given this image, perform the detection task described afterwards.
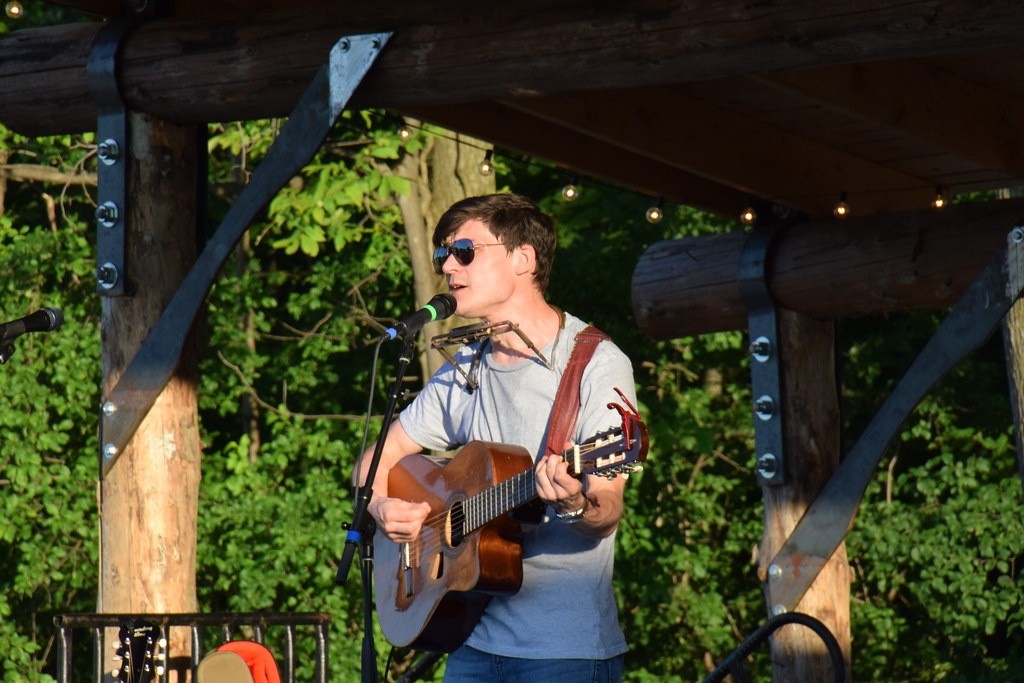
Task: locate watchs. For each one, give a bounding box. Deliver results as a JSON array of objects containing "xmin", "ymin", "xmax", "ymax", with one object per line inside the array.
[{"xmin": 554, "ymin": 499, "xmax": 590, "ymax": 525}]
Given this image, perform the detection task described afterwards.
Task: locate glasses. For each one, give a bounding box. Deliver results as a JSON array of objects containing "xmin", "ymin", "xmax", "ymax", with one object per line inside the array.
[{"xmin": 432, "ymin": 238, "xmax": 515, "ymax": 275}]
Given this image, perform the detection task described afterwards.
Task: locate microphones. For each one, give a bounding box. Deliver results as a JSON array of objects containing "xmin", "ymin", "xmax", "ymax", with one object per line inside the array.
[
  {"xmin": 1, "ymin": 306, "xmax": 64, "ymax": 347},
  {"xmin": 335, "ymin": 529, "xmax": 361, "ymax": 586},
  {"xmin": 381, "ymin": 292, "xmax": 457, "ymax": 342}
]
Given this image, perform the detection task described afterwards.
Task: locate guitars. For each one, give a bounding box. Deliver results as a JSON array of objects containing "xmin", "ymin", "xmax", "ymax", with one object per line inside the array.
[{"xmin": 374, "ymin": 387, "xmax": 650, "ymax": 653}]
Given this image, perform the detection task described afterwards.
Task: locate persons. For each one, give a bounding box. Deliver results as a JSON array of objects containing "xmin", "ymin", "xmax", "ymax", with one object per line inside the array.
[{"xmin": 353, "ymin": 193, "xmax": 638, "ymax": 681}]
